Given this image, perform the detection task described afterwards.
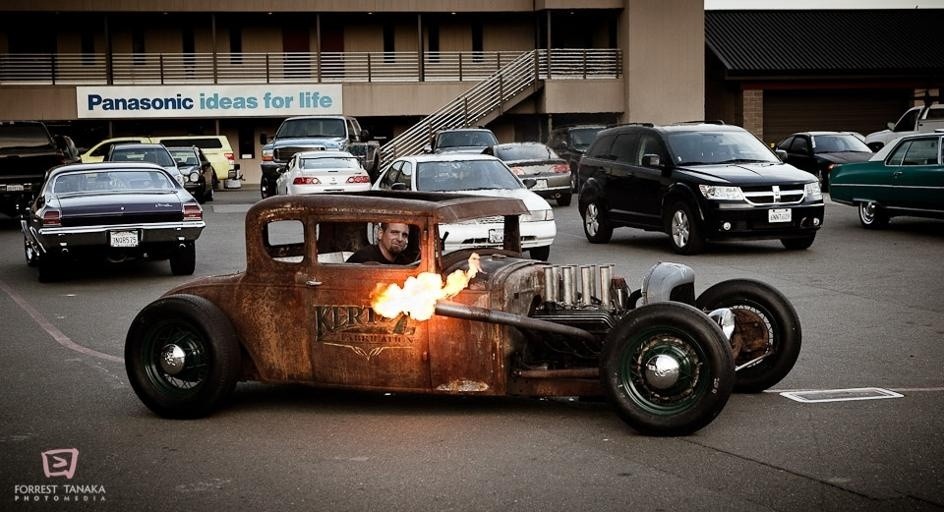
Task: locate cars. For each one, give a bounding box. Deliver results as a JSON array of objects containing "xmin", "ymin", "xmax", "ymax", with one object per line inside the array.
[
  {"xmin": 825, "ymin": 126, "xmax": 939, "ymax": 237},
  {"xmin": 479, "ymin": 141, "xmax": 576, "ymax": 209},
  {"xmin": 102, "ymin": 142, "xmax": 186, "ymax": 206},
  {"xmin": 773, "ymin": 132, "xmax": 878, "ymax": 194},
  {"xmin": 364, "ymin": 147, "xmax": 561, "ymax": 264},
  {"xmin": 164, "ymin": 146, "xmax": 214, "ymax": 203},
  {"xmin": 274, "ymin": 151, "xmax": 374, "ymax": 210},
  {"xmin": 123, "ymin": 189, "xmax": 807, "ymax": 442},
  {"xmin": 17, "ymin": 152, "xmax": 209, "ymax": 298},
  {"xmin": 422, "ymin": 128, "xmax": 499, "ymax": 157}
]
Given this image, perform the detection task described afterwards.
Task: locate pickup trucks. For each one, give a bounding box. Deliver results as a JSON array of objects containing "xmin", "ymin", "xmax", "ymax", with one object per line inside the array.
[
  {"xmin": 260, "ymin": 110, "xmax": 382, "ymax": 200},
  {"xmin": 81, "ymin": 133, "xmax": 242, "ymax": 189}
]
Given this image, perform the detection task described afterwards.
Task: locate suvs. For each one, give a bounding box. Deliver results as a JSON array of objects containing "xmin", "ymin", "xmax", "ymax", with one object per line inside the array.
[
  {"xmin": 0, "ymin": 118, "xmax": 69, "ymax": 222},
  {"xmin": 571, "ymin": 118, "xmax": 824, "ymax": 256},
  {"xmin": 864, "ymin": 100, "xmax": 943, "ymax": 159},
  {"xmin": 546, "ymin": 126, "xmax": 608, "ymax": 191}
]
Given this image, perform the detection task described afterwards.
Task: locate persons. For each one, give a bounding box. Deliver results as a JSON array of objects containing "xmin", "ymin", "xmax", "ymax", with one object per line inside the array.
[{"xmin": 345, "ymin": 222, "xmax": 411, "ymax": 264}]
